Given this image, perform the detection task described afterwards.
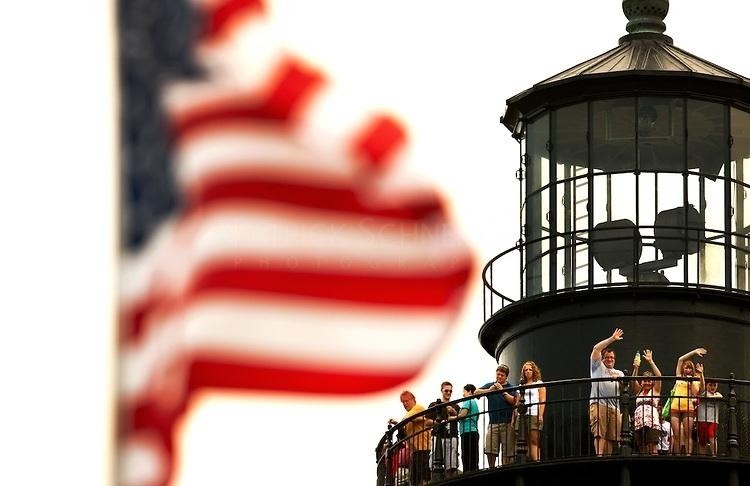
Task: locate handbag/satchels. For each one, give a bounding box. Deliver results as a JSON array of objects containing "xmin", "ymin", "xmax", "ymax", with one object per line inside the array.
[
  {"xmin": 628, "ymin": 387, "xmax": 644, "ymax": 413},
  {"xmin": 661, "ymin": 384, "xmax": 677, "ymax": 418}
]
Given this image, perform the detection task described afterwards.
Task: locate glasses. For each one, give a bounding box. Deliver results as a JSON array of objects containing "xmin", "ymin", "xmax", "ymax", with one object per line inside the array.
[{"xmin": 443, "ymin": 390, "xmax": 453, "ymax": 393}]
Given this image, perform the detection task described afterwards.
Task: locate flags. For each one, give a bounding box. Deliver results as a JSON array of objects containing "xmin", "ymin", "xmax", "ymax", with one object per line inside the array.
[{"xmin": 111, "ymin": 0, "xmax": 481, "ymax": 486}]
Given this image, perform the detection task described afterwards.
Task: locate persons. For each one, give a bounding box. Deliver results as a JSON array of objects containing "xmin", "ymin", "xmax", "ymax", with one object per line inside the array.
[
  {"xmin": 588, "ymin": 327, "xmax": 627, "ymax": 459},
  {"xmin": 662, "ymin": 347, "xmax": 708, "ymax": 457},
  {"xmin": 387, "ymin": 390, "xmax": 429, "ymax": 486},
  {"xmin": 695, "ymin": 363, "xmax": 724, "ymax": 458},
  {"xmin": 448, "ymin": 383, "xmax": 484, "ymax": 473},
  {"xmin": 629, "ymin": 349, "xmax": 663, "ymax": 458},
  {"xmin": 509, "ymin": 360, "xmax": 545, "ymax": 465},
  {"xmin": 426, "ymin": 380, "xmax": 462, "ymax": 479},
  {"xmin": 472, "ymin": 364, "xmax": 517, "ymax": 467},
  {"xmin": 657, "ymin": 404, "xmax": 676, "ymax": 457}
]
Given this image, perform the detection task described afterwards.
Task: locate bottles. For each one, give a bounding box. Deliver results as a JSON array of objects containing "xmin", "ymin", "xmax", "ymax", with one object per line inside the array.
[{"xmin": 633, "ymin": 349, "xmax": 641, "ymax": 366}]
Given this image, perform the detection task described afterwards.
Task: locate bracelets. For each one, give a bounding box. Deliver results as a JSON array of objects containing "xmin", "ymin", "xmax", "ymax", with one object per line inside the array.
[{"xmin": 503, "ymin": 392, "xmax": 508, "ymax": 397}]
